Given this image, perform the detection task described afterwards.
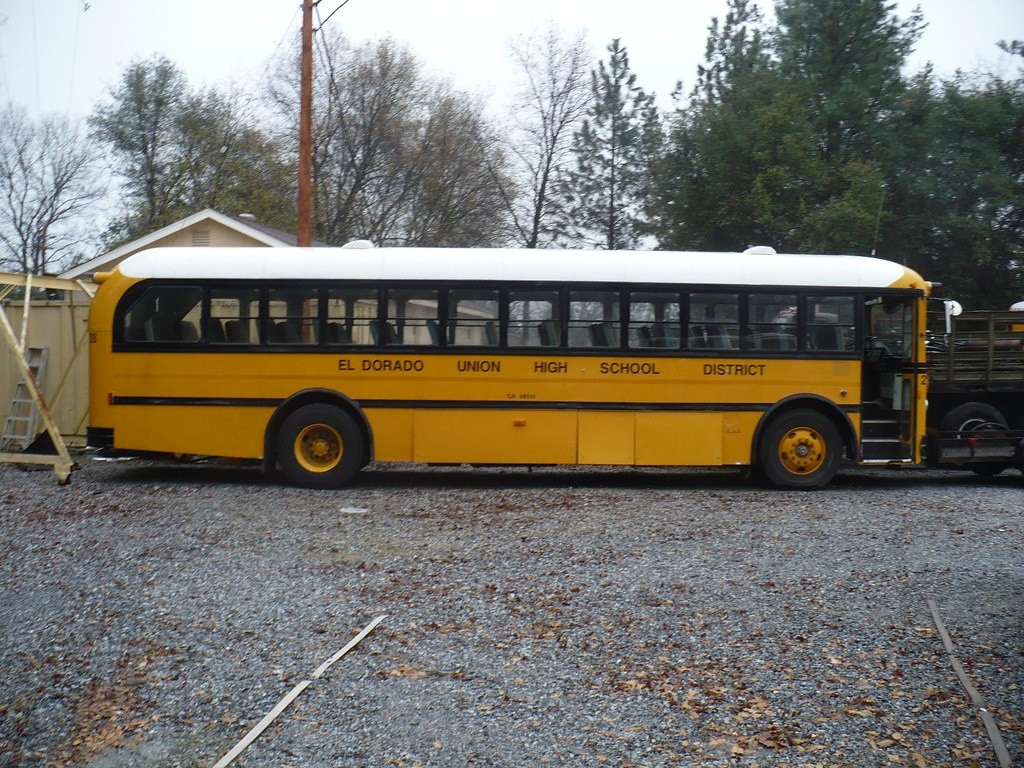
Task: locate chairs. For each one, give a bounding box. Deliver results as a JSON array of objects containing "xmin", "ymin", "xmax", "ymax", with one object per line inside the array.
[
  {"xmin": 329, "ymin": 322, "xmax": 354, "ymax": 344},
  {"xmin": 226, "ymin": 320, "xmax": 248, "ymax": 343},
  {"xmin": 255, "ymin": 317, "xmax": 274, "ymax": 344},
  {"xmin": 538, "ymin": 324, "xmax": 551, "ymax": 346},
  {"xmin": 542, "ymin": 320, "xmax": 559, "ymax": 345},
  {"xmin": 636, "ymin": 325, "xmax": 652, "ymax": 347},
  {"xmin": 312, "ymin": 318, "xmax": 330, "ymax": 344},
  {"xmin": 485, "ymin": 322, "xmax": 497, "ymax": 344},
  {"xmin": 176, "ymin": 321, "xmax": 199, "ymax": 341},
  {"xmin": 707, "ymin": 324, "xmax": 733, "ymax": 348},
  {"xmin": 273, "ymin": 323, "xmax": 301, "ymax": 343},
  {"xmin": 427, "ymin": 320, "xmax": 440, "ymax": 344},
  {"xmin": 600, "ymin": 322, "xmax": 619, "ymax": 346},
  {"xmin": 784, "ymin": 327, "xmax": 814, "ymax": 350},
  {"xmin": 369, "ymin": 321, "xmax": 399, "ymax": 345},
  {"xmin": 691, "ymin": 326, "xmax": 705, "ymax": 348},
  {"xmin": 587, "ymin": 323, "xmax": 601, "ymax": 346},
  {"xmin": 148, "ymin": 316, "xmax": 178, "ymax": 341},
  {"xmin": 757, "ymin": 326, "xmax": 780, "ymax": 349},
  {"xmin": 652, "ymin": 322, "xmax": 674, "ymax": 348},
  {"xmin": 816, "ymin": 326, "xmax": 843, "ymax": 350},
  {"xmin": 742, "ymin": 327, "xmax": 756, "ymax": 349},
  {"xmin": 200, "ymin": 317, "xmax": 225, "ymax": 343}
]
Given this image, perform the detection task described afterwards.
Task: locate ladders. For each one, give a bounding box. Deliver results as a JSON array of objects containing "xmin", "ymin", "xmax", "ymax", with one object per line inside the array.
[{"xmin": 0, "ymin": 346, "xmax": 49, "ymax": 450}]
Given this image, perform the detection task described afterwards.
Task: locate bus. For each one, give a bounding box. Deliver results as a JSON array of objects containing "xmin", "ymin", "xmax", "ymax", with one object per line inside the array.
[{"xmin": 88, "ymin": 240, "xmax": 953, "ymax": 491}]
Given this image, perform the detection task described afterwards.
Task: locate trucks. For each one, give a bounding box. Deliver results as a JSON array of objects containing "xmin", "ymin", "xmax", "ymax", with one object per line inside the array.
[{"xmin": 772, "ymin": 298, "xmax": 1024, "ymax": 476}]
